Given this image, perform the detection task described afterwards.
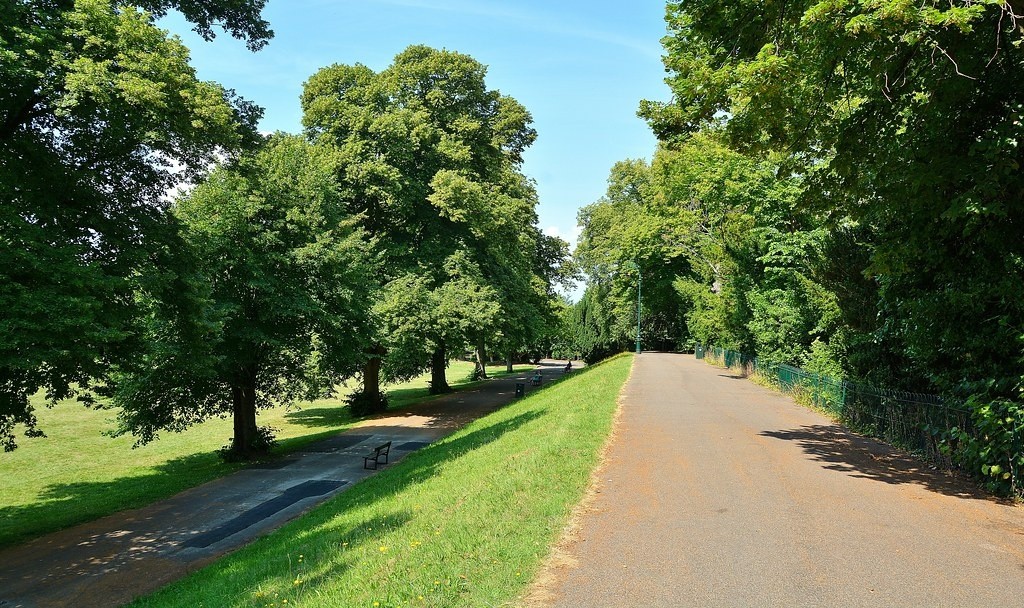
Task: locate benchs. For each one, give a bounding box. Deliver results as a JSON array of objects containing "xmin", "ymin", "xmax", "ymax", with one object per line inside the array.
[{"xmin": 363, "ymin": 441, "xmax": 392, "ymax": 470}]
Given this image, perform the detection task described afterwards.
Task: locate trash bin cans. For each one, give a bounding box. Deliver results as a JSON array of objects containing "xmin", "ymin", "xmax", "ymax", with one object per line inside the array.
[
  {"xmin": 695, "ymin": 342, "xmax": 704, "ymax": 359},
  {"xmin": 515, "ymin": 383, "xmax": 525, "ymax": 398}
]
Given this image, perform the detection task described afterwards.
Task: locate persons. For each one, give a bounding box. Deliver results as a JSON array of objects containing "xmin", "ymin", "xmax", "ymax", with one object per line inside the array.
[
  {"xmin": 527, "ymin": 370, "xmax": 542, "ymax": 386},
  {"xmin": 564, "ymin": 360, "xmax": 572, "ymax": 374}
]
